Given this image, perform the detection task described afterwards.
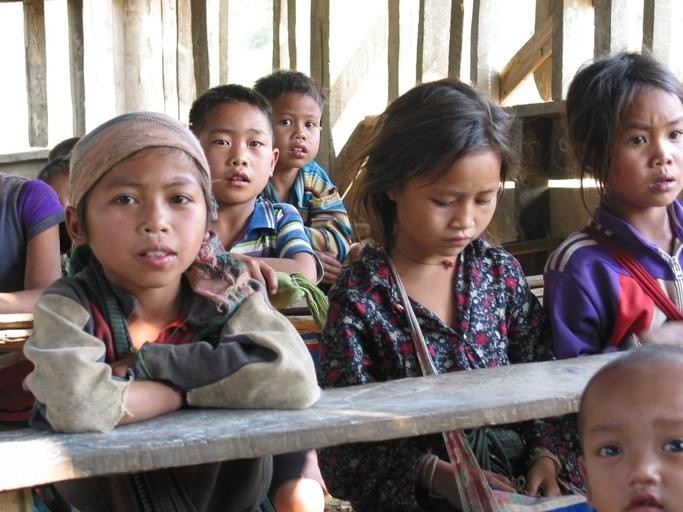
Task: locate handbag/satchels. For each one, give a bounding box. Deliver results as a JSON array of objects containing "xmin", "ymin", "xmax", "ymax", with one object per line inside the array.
[{"xmin": 458, "ymin": 480, "xmax": 589, "ymax": 510}]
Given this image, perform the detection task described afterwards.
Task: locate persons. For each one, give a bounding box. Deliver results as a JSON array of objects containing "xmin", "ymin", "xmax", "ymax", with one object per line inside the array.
[
  {"xmin": 257, "ymin": 70, "xmax": 352, "ymax": 285},
  {"xmin": 574, "ymin": 348, "xmax": 682, "ymax": 510},
  {"xmin": 0, "ymin": 171, "xmax": 61, "ymax": 315},
  {"xmin": 35, "ymin": 159, "xmax": 74, "ymax": 216},
  {"xmin": 22, "ymin": 112, "xmax": 329, "ymax": 512},
  {"xmin": 319, "ymin": 76, "xmax": 579, "ymax": 510},
  {"xmin": 543, "ymin": 51, "xmax": 682, "ymax": 352},
  {"xmin": 191, "ymin": 87, "xmax": 324, "ymax": 294}
]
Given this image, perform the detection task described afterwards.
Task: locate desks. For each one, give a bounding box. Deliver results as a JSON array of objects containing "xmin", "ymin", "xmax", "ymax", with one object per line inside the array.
[{"xmin": 0, "ymin": 347, "xmax": 628, "ymax": 491}]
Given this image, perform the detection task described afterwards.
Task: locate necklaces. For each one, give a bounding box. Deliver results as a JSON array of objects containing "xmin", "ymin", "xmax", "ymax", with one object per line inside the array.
[{"xmin": 393, "ymin": 255, "xmax": 464, "ymax": 270}]
